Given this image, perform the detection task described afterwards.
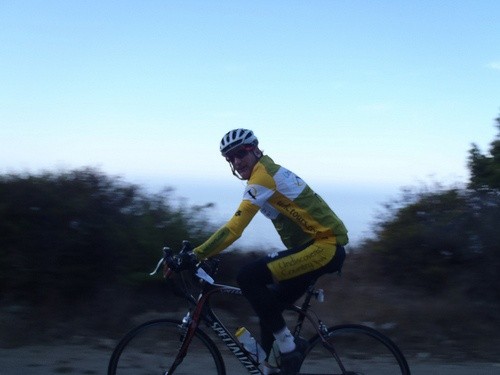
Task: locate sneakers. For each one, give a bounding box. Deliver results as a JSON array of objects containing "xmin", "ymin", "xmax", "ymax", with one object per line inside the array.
[{"xmin": 278, "ymin": 334, "xmax": 311, "ymax": 375}]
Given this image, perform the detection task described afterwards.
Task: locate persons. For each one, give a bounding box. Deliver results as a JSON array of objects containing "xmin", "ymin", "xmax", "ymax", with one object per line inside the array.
[{"xmin": 162, "ymin": 128, "xmax": 350, "ymax": 375}]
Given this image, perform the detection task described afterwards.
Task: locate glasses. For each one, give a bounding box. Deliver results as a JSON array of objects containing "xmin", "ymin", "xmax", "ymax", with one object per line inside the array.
[{"xmin": 226, "ymin": 148, "xmax": 253, "ymax": 162}]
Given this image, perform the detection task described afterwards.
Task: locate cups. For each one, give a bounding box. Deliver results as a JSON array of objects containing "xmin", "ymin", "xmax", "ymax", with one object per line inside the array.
[{"xmin": 235, "ymin": 326, "xmax": 267, "ymax": 363}]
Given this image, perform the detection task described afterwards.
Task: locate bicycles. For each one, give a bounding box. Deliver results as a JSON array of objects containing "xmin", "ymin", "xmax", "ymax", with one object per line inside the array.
[{"xmin": 108, "ymin": 240, "xmax": 413, "ymax": 375}]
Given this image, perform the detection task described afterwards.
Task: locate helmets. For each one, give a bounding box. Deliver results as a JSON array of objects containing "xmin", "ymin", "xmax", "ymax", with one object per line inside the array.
[{"xmin": 220, "ymin": 129, "xmax": 258, "ymax": 156}]
